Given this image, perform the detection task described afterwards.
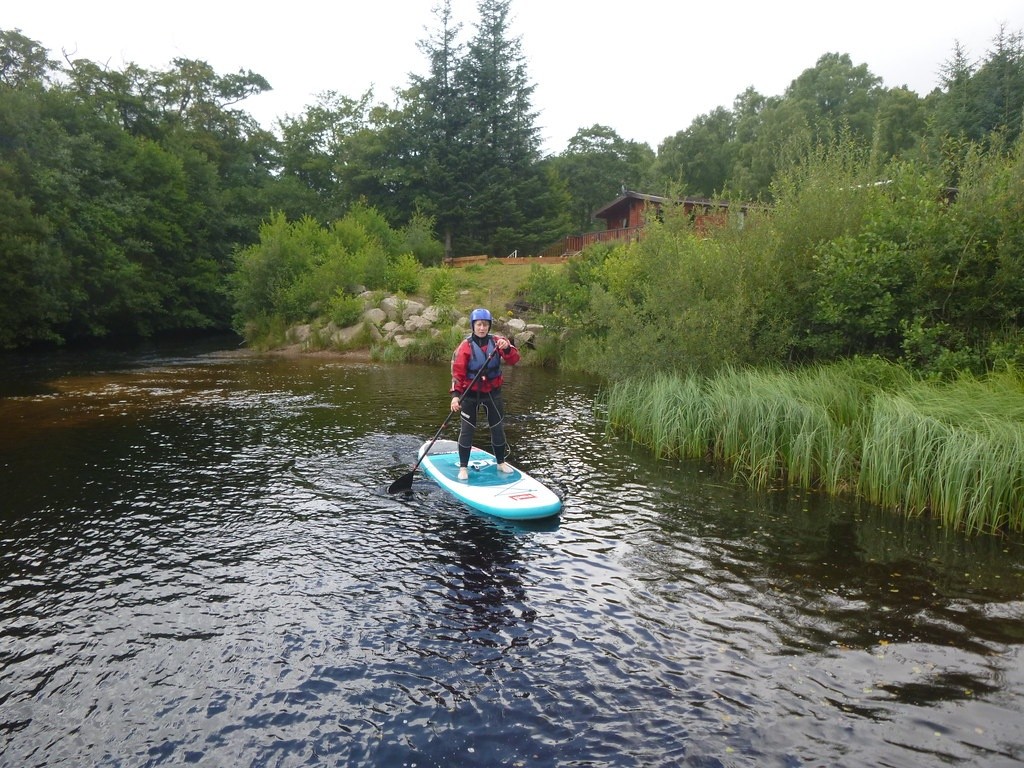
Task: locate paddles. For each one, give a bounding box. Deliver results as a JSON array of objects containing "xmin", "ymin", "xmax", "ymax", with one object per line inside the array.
[{"xmin": 387, "ymin": 344, "xmax": 500, "ymax": 495}]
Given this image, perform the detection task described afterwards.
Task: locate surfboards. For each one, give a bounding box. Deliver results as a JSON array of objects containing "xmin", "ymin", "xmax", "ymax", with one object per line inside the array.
[{"xmin": 420, "ymin": 437, "xmax": 562, "ymax": 519}]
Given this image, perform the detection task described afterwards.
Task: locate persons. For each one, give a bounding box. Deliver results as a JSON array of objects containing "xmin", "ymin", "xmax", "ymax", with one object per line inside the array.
[{"xmin": 450, "ymin": 309, "xmax": 521, "ymax": 480}]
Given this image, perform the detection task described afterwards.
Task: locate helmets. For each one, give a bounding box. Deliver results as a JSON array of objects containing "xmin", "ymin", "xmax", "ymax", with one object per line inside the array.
[{"xmin": 470, "ymin": 309, "xmax": 492, "ymax": 331}]
